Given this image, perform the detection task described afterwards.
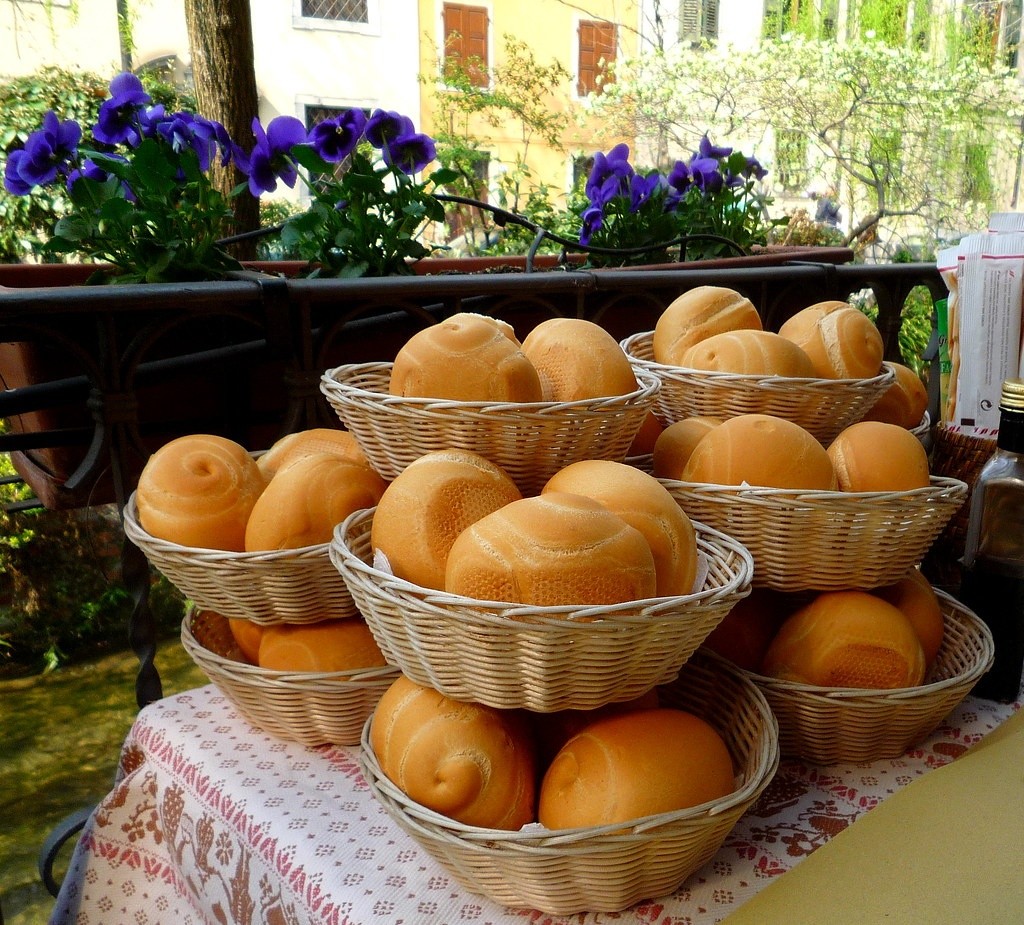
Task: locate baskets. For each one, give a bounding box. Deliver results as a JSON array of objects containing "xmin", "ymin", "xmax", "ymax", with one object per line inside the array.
[{"xmin": 120, "ymin": 331, "xmax": 993, "ymax": 915}]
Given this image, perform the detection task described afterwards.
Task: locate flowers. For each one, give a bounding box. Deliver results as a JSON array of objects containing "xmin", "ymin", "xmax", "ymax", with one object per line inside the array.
[{"xmin": 0, "ymin": 72, "xmax": 790, "ymax": 291}]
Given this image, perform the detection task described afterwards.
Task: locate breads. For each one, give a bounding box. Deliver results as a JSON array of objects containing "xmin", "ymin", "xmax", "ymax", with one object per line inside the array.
[{"xmin": 137, "ymin": 286, "xmax": 942, "ymax": 832}]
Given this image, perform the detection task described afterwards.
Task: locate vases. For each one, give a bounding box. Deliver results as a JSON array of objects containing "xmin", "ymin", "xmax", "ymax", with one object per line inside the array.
[{"xmin": 0, "ymin": 245, "xmax": 855, "ymax": 510}]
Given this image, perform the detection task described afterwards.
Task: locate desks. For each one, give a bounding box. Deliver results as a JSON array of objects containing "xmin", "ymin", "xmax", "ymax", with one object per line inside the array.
[{"xmin": 49, "ymin": 682, "xmax": 1023, "ymax": 925}]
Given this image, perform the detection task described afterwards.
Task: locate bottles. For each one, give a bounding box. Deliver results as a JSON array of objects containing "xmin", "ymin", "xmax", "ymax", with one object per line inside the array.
[{"xmin": 964, "ymin": 380, "xmax": 1024, "ymax": 578}]
